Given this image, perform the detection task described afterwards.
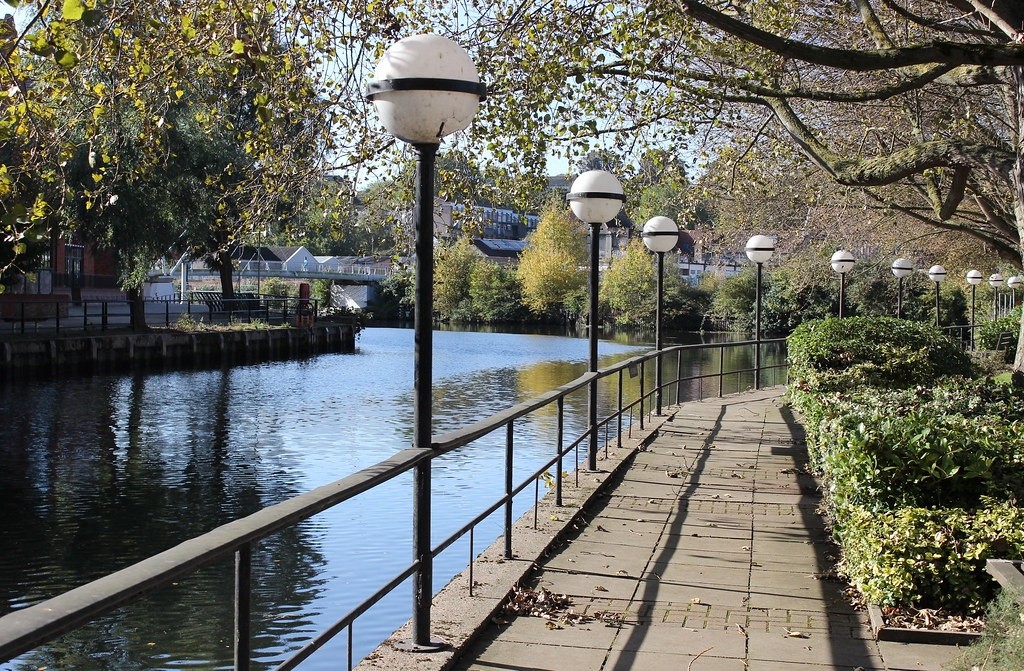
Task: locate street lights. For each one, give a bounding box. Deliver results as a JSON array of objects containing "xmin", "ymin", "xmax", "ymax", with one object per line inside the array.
[
  {"xmin": 640, "ymin": 216, "xmax": 680, "ymax": 416},
  {"xmin": 1007, "ymin": 277, "xmax": 1021, "ymax": 308},
  {"xmin": 566, "ymin": 169, "xmax": 626, "ymax": 473},
  {"xmin": 744, "ymin": 236, "xmax": 776, "ymax": 391},
  {"xmin": 989, "ymin": 273, "xmax": 1004, "ymax": 321},
  {"xmin": 929, "ymin": 265, "xmax": 947, "ymax": 331},
  {"xmin": 890, "ymin": 258, "xmax": 913, "ymax": 320},
  {"xmin": 966, "ymin": 270, "xmax": 983, "ymax": 351},
  {"xmin": 366, "ymin": 34, "xmax": 488, "ymax": 652},
  {"xmin": 831, "ymin": 250, "xmax": 857, "ymax": 319}
]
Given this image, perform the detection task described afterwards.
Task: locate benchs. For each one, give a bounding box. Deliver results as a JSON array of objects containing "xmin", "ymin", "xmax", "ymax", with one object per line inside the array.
[{"xmin": 199, "ymin": 291, "xmax": 269, "ymax": 325}]
